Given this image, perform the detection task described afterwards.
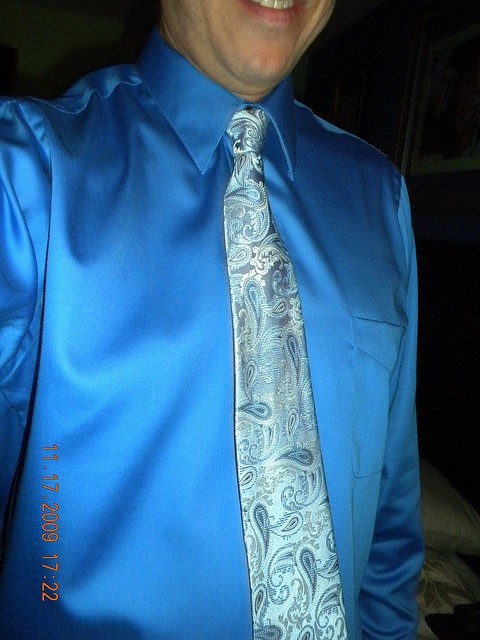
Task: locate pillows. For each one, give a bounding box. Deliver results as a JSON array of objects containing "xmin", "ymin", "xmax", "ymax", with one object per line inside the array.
[{"xmin": 419, "ymin": 457, "xmax": 480, "ymax": 555}]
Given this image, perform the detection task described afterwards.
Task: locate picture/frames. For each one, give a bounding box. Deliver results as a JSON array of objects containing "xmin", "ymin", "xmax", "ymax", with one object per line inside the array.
[{"xmin": 392, "ymin": 0, "xmax": 480, "ymax": 216}]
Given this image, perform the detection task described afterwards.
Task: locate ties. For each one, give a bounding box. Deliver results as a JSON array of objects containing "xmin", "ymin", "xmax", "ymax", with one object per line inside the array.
[{"xmin": 224, "ymin": 109, "xmax": 346, "ymax": 640}]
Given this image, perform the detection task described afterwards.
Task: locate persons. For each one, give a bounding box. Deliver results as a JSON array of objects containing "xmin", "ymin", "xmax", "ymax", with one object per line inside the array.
[
  {"xmin": 422, "ymin": 36, "xmax": 480, "ymax": 160},
  {"xmin": 1, "ymin": 1, "xmax": 480, "ymax": 639}
]
